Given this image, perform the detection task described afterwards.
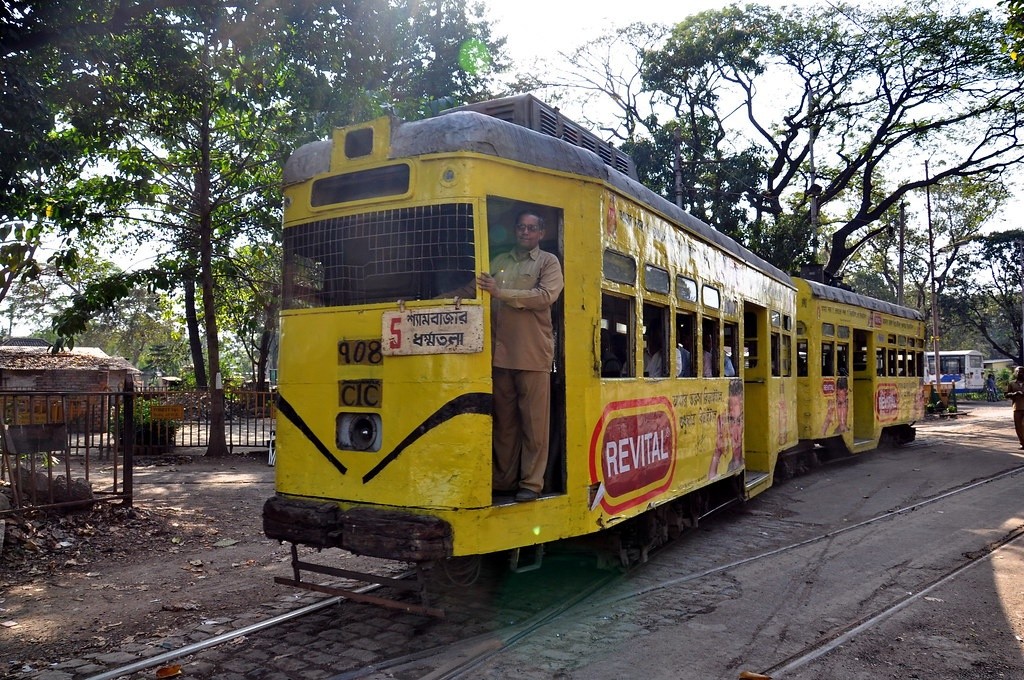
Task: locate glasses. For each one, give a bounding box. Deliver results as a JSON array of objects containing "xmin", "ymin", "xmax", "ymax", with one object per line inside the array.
[{"xmin": 516, "ymin": 224, "xmax": 537, "ymax": 231}]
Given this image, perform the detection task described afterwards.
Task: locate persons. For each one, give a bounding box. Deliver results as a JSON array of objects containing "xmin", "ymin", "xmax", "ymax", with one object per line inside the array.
[
  {"xmin": 1003, "ymin": 366, "xmax": 1024, "ymax": 450},
  {"xmin": 986, "ymin": 374, "xmax": 1001, "ymax": 402},
  {"xmin": 797, "ymin": 343, "xmax": 849, "ymax": 376},
  {"xmin": 601, "ymin": 317, "xmax": 735, "ymax": 377},
  {"xmin": 431, "ymin": 213, "xmax": 562, "ymax": 503},
  {"xmin": 899, "ymin": 360, "xmax": 923, "ymax": 377}
]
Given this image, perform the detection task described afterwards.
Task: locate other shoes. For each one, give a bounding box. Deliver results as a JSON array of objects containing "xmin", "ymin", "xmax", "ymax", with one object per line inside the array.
[
  {"xmin": 514, "ymin": 488, "xmax": 539, "ymax": 501},
  {"xmin": 492, "ymin": 490, "xmax": 517, "ymax": 497},
  {"xmin": 1018, "ymin": 444, "xmax": 1024, "ymax": 450}
]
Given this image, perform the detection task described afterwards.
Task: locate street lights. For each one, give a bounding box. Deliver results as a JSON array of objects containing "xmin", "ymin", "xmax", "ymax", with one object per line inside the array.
[{"xmin": 929, "ymin": 239, "xmax": 969, "ymax": 397}]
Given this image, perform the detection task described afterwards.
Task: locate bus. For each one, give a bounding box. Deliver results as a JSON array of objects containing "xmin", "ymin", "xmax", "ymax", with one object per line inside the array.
[
  {"xmin": 263, "ymin": 93, "xmax": 927, "ymax": 619},
  {"xmin": 882, "ymin": 350, "xmax": 985, "ymax": 393}
]
